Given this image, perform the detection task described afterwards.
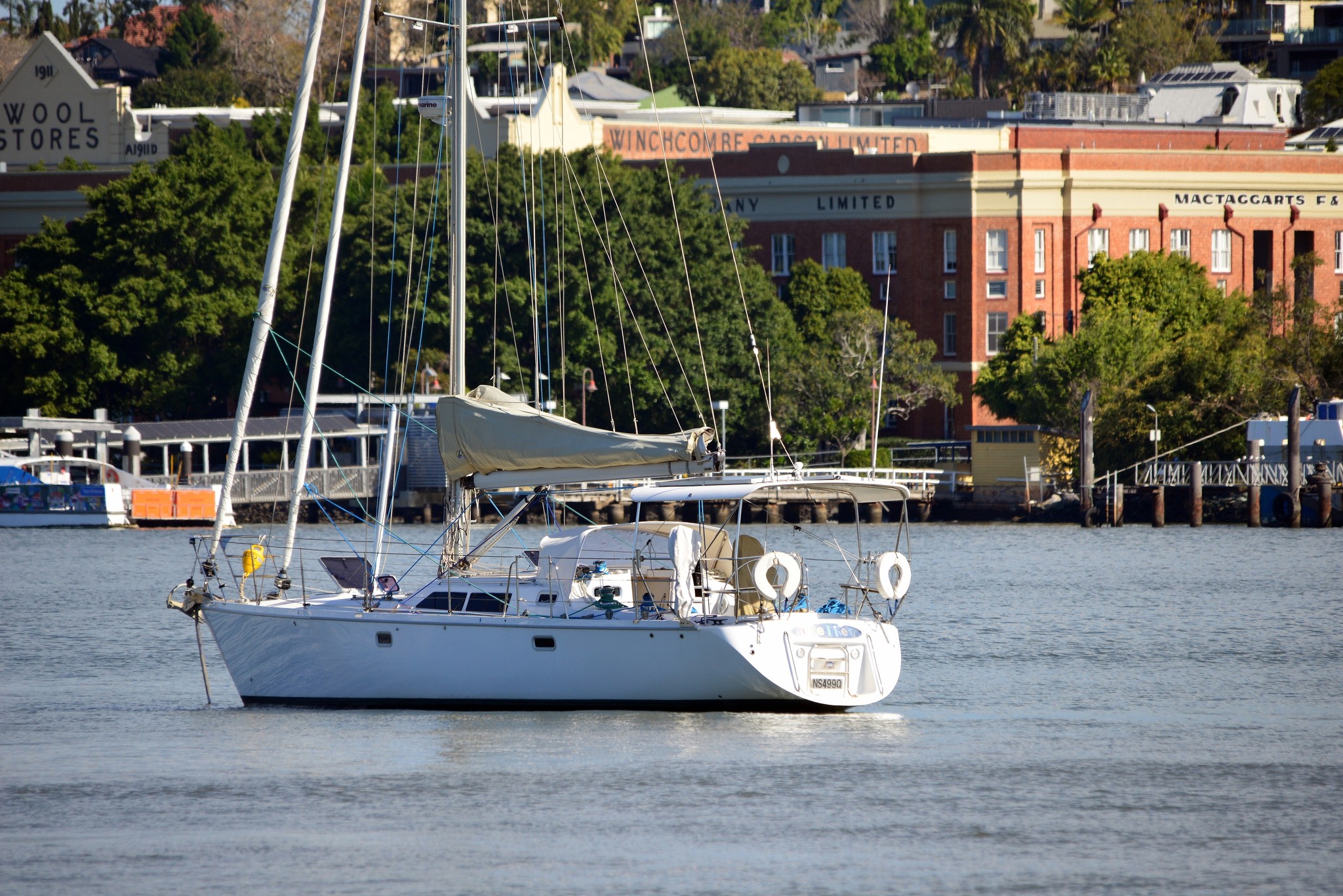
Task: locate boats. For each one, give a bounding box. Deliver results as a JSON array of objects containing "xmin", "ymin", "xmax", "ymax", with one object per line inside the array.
[{"xmin": 0, "ymin": 451, "xmax": 129, "ymax": 528}]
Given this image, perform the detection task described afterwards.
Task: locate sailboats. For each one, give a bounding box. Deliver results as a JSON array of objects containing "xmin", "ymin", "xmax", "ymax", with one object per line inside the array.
[{"xmin": 163, "ymin": 0, "xmax": 912, "ymax": 715}]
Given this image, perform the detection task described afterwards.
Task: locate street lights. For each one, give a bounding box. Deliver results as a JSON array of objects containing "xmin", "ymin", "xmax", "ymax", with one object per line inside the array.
[
  {"xmin": 534, "ymin": 366, "xmax": 549, "ymax": 410},
  {"xmin": 421, "ymin": 359, "xmax": 443, "ymax": 396},
  {"xmin": 710, "ymin": 400, "xmax": 729, "ymax": 477},
  {"xmin": 1147, "ymin": 404, "xmax": 1157, "ymax": 485},
  {"xmin": 582, "ymin": 367, "xmax": 598, "ymax": 425},
  {"xmin": 868, "ymin": 365, "xmax": 880, "ymax": 468},
  {"xmin": 497, "ymin": 366, "xmax": 511, "ymax": 391}
]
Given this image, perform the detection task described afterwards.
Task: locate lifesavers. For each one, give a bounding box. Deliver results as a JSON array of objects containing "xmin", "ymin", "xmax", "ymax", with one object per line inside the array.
[
  {"xmin": 106, "ymin": 469, "xmax": 119, "ymax": 484},
  {"xmin": 752, "ymin": 551, "xmax": 801, "ymax": 601},
  {"xmin": 1082, "ymin": 507, "xmax": 1103, "ymax": 528},
  {"xmin": 875, "ymin": 552, "xmax": 911, "ymax": 599},
  {"xmin": 1273, "ymin": 492, "xmax": 1302, "ymax": 522}
]
